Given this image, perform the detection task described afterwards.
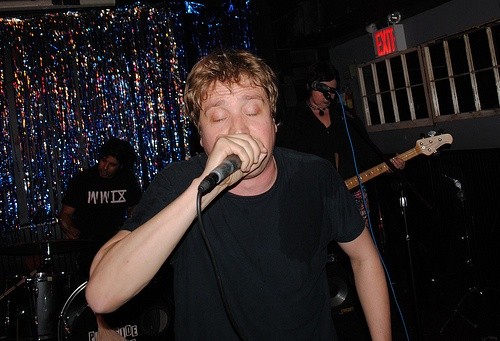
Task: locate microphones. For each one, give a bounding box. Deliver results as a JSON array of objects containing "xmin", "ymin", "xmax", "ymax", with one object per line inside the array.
[
  {"xmin": 198, "ymin": 154, "xmax": 242, "ymax": 193},
  {"xmin": 19, "ymin": 216, "xmax": 59, "ymax": 230},
  {"xmin": 311, "ymin": 81, "xmax": 336, "ymax": 94}
]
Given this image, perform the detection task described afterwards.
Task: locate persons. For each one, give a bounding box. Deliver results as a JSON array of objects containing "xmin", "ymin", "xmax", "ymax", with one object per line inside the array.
[
  {"xmin": 279, "ymin": 62, "xmax": 406, "ymax": 315},
  {"xmin": 85, "ymin": 49, "xmax": 394, "ymax": 341},
  {"xmin": 57, "ymin": 138, "xmax": 144, "ymax": 266}
]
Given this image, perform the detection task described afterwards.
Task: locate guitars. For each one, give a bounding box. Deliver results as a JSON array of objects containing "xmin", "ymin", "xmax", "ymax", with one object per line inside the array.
[{"xmin": 343, "ymin": 128, "xmax": 454, "ymax": 191}]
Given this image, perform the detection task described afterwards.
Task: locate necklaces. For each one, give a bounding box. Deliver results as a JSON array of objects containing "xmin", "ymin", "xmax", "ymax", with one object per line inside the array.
[{"xmin": 307, "ymin": 100, "xmax": 328, "ymax": 116}]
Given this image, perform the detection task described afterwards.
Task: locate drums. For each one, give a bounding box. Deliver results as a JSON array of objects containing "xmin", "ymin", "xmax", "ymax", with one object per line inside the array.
[
  {"xmin": 57, "ymin": 280, "xmax": 173, "ymax": 341},
  {"xmin": 0, "ymin": 274, "xmax": 63, "ymax": 341}
]
